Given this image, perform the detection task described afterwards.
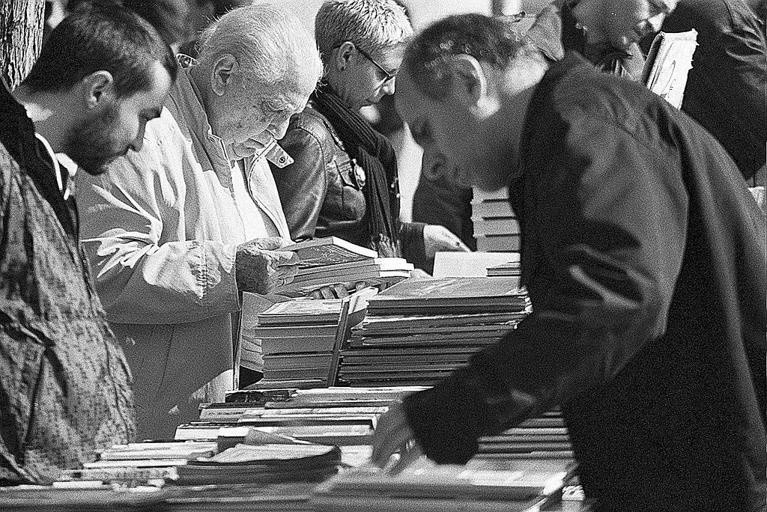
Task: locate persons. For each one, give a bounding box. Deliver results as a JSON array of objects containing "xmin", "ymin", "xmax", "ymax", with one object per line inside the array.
[
  {"xmin": 640, "ymin": 0, "xmax": 766, "ymax": 208},
  {"xmin": 407, "ymin": 0, "xmax": 679, "ymax": 276},
  {"xmin": 76, "ymin": 4, "xmax": 324, "ymax": 437},
  {"xmin": 372, "ymin": 13, "xmax": 764, "ymax": 512},
  {"xmin": 239, "ymin": 0, "xmax": 471, "ymax": 384},
  {"xmin": 0, "ymin": 6, "xmax": 180, "ymax": 485}
]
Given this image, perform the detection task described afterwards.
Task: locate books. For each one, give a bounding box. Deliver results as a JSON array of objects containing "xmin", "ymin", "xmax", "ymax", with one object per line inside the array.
[{"xmin": 1, "ymin": 189, "xmax": 594, "ymax": 512}]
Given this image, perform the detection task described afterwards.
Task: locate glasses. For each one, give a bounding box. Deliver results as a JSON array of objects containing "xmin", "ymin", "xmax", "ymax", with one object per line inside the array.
[{"xmin": 351, "ymin": 44, "xmax": 399, "ymax": 84}]
